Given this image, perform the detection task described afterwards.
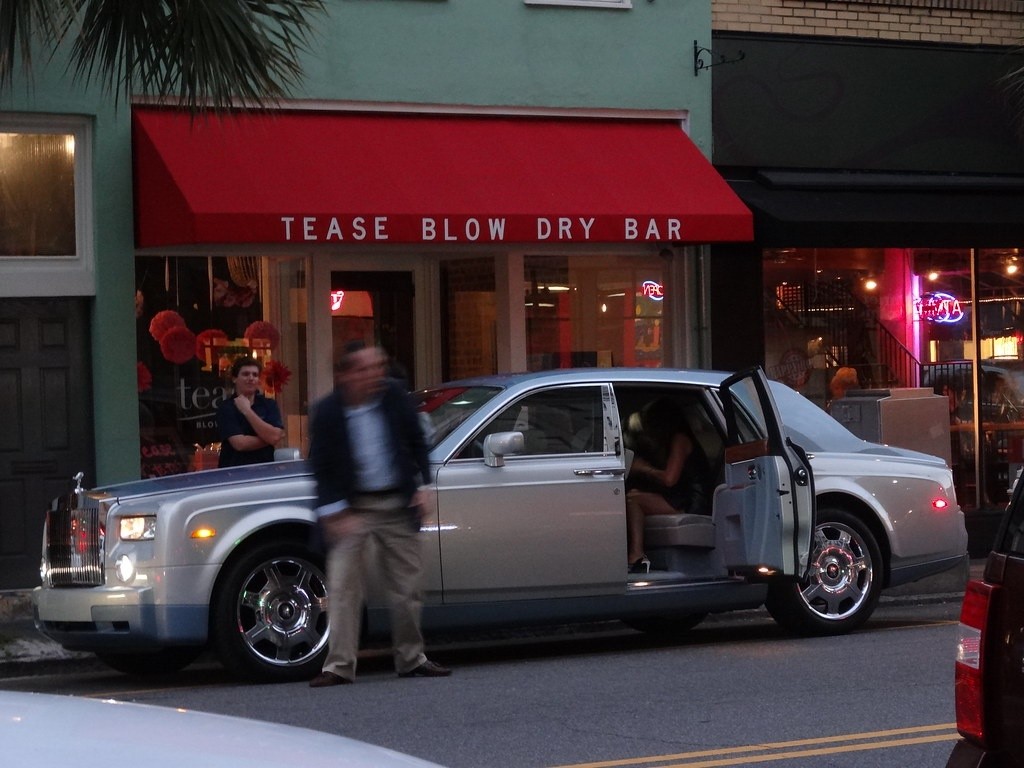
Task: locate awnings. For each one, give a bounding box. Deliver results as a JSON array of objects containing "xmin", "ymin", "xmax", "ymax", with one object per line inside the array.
[
  {"xmin": 730, "ymin": 185, "xmax": 1024, "ymax": 248},
  {"xmin": 132, "ymin": 108, "xmax": 754, "ymax": 246}
]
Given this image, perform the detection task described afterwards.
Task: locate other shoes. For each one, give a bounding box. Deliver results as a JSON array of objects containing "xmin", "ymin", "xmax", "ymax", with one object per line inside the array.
[
  {"xmin": 309, "ymin": 671, "xmax": 353, "ymax": 687},
  {"xmin": 399, "ymin": 660, "xmax": 452, "ymax": 677}
]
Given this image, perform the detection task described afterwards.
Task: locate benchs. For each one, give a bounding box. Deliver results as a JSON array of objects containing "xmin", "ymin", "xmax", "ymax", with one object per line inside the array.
[{"xmin": 642, "ymin": 512, "xmax": 715, "ymax": 551}]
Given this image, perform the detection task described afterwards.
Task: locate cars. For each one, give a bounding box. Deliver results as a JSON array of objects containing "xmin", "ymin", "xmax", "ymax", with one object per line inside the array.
[
  {"xmin": 926, "ymin": 363, "xmax": 1024, "ymax": 423},
  {"xmin": 0, "ymin": 689, "xmax": 440, "ymax": 767},
  {"xmin": 937, "ymin": 467, "xmax": 1024, "ymax": 763},
  {"xmin": 28, "ymin": 365, "xmax": 970, "ymax": 676}
]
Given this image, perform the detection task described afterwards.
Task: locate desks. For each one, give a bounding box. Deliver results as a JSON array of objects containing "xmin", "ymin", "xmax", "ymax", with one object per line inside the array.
[{"xmin": 950, "ymin": 421, "xmax": 1024, "ymax": 512}]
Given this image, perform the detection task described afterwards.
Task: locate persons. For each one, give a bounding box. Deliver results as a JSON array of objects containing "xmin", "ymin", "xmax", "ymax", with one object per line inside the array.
[
  {"xmin": 306, "ymin": 340, "xmax": 452, "ymax": 687},
  {"xmin": 627, "ymin": 402, "xmax": 715, "ymax": 574},
  {"xmin": 941, "ymin": 369, "xmax": 1017, "ymax": 504},
  {"xmin": 218, "ymin": 357, "xmax": 285, "ymax": 467},
  {"xmin": 831, "ymin": 367, "xmax": 857, "ymax": 397}
]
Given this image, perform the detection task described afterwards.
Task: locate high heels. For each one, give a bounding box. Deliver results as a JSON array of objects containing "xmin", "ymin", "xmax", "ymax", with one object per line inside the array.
[{"xmin": 628, "ymin": 554, "xmax": 650, "ymax": 574}]
